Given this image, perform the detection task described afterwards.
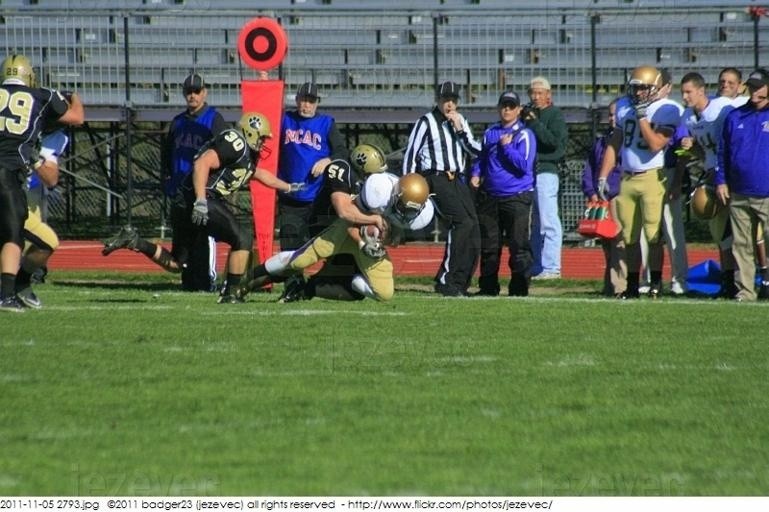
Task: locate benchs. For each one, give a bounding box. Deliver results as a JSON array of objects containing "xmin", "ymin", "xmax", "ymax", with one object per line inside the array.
[{"xmin": 0, "ymin": 0, "xmax": 769, "ymax": 112}]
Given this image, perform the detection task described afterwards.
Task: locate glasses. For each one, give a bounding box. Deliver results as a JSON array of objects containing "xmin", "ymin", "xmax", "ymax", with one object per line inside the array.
[
  {"xmin": 184, "ymin": 87, "xmax": 203, "ymax": 95},
  {"xmin": 501, "ymin": 102, "xmax": 517, "ymax": 110}
]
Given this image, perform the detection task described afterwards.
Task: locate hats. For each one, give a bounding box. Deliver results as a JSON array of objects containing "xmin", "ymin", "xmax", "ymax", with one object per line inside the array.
[
  {"xmin": 182, "ymin": 73, "xmax": 206, "ymax": 90},
  {"xmin": 527, "ymin": 76, "xmax": 550, "ymax": 91},
  {"xmin": 742, "ymin": 68, "xmax": 767, "ymax": 90},
  {"xmin": 296, "ymin": 82, "xmax": 318, "ymax": 98},
  {"xmin": 498, "ymin": 91, "xmax": 519, "ymax": 106},
  {"xmin": 436, "ymin": 81, "xmax": 461, "ymax": 99}
]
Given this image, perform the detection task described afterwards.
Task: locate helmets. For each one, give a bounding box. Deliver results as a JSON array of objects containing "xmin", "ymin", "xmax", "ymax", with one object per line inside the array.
[
  {"xmin": 691, "ymin": 183, "xmax": 724, "ymax": 221},
  {"xmin": 349, "ymin": 142, "xmax": 430, "ymax": 222},
  {"xmin": 235, "ymin": 110, "xmax": 273, "ymax": 152},
  {"xmin": 0, "ymin": 52, "xmax": 37, "ymax": 88},
  {"xmin": 624, "ymin": 65, "xmax": 664, "ymax": 103}
]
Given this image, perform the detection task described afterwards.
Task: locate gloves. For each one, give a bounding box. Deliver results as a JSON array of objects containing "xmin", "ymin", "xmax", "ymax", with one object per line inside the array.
[
  {"xmin": 359, "ymin": 239, "xmax": 387, "ymax": 259},
  {"xmin": 191, "ymin": 198, "xmax": 209, "ymax": 226}
]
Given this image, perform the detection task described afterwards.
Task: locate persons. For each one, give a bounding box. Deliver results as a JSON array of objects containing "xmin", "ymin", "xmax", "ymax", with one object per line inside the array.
[
  {"xmin": 582, "ymin": 67, "xmax": 768, "ymax": 301},
  {"xmin": 102, "ymin": 72, "xmax": 569, "ymax": 301},
  {"xmin": 0, "ymin": 54, "xmax": 84, "ymax": 312}
]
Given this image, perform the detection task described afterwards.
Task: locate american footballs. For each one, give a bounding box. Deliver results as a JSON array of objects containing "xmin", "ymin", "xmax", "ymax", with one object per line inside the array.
[{"xmin": 360, "ymin": 224, "xmax": 386, "ymax": 240}]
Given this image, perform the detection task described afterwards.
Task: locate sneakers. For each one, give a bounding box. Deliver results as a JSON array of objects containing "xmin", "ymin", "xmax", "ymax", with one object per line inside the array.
[
  {"xmin": 100, "ymin": 222, "xmax": 140, "ymax": 257},
  {"xmin": 618, "ymin": 272, "xmax": 769, "ymax": 302},
  {"xmin": 216, "ymin": 271, "xmax": 317, "ymax": 305},
  {"xmin": 1, "ymin": 285, "xmax": 40, "ymax": 312}
]
